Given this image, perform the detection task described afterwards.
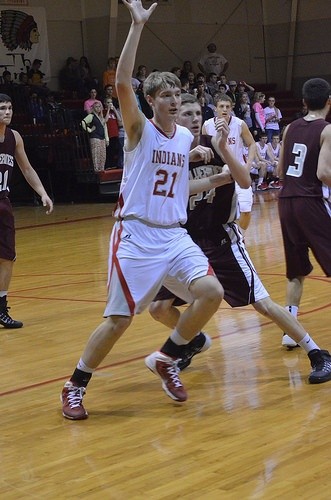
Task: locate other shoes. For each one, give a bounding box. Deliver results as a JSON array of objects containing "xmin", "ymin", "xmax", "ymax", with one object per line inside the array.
[{"xmin": 252, "ymin": 180, "xmax": 282, "ymax": 192}]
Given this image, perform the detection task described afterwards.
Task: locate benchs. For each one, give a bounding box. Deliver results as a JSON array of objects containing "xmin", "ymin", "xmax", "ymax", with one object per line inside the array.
[
  {"xmin": 247, "ymin": 83, "xmax": 303, "ymax": 125},
  {"xmin": 14, "ymin": 89, "xmax": 85, "ymax": 163}
]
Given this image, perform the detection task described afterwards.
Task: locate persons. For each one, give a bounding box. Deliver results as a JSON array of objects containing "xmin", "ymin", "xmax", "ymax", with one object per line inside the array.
[
  {"xmin": 148, "ymin": 94, "xmax": 331, "ymax": 385},
  {"xmin": 3, "ymin": 41, "xmax": 310, "ymax": 192},
  {"xmin": 202, "ymin": 95, "xmax": 258, "ymax": 230},
  {"xmin": 61, "ymin": 0, "xmax": 254, "ymax": 421},
  {"xmin": 0, "ymin": 94, "xmax": 53, "ymax": 330},
  {"xmin": 277, "ymin": 78, "xmax": 331, "ymax": 348}
]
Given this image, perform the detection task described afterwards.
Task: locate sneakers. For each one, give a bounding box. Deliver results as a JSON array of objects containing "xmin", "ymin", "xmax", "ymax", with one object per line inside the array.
[
  {"xmin": 144, "ymin": 350, "xmax": 187, "ymax": 402},
  {"xmin": 306, "ymin": 348, "xmax": 331, "ymax": 384},
  {"xmin": 0, "ymin": 301, "xmax": 23, "ymax": 328},
  {"xmin": 177, "ymin": 331, "xmax": 211, "ymax": 371},
  {"xmin": 60, "ymin": 379, "xmax": 88, "ymax": 420},
  {"xmin": 281, "ymin": 331, "xmax": 299, "ymax": 347}
]
